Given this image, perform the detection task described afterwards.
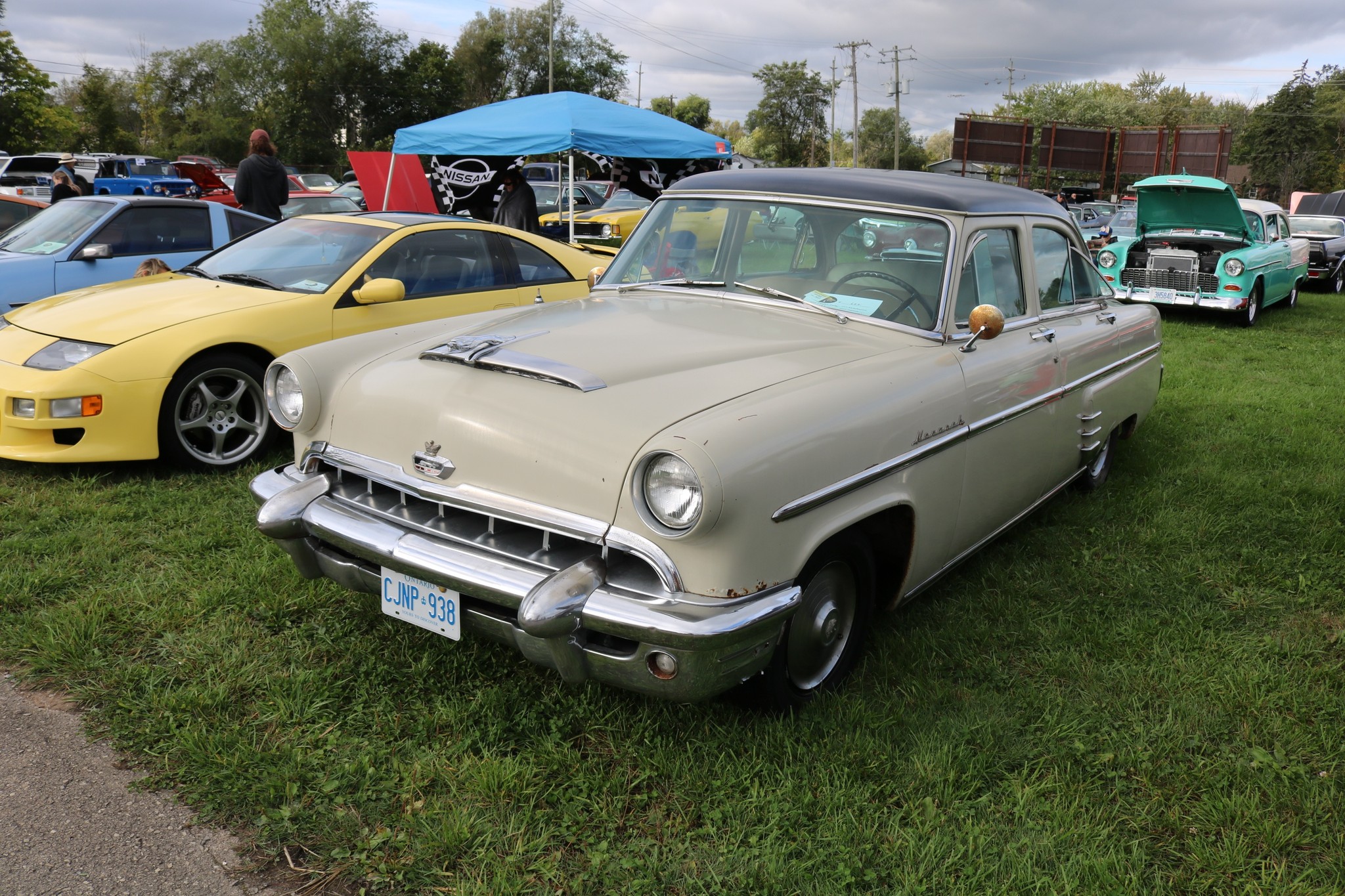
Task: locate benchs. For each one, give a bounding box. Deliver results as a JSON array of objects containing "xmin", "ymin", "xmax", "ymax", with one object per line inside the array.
[
  {"xmin": 733, "ymin": 276, "xmax": 931, "ymax": 328},
  {"xmin": 826, "ymin": 262, "xmax": 976, "ymax": 320}
]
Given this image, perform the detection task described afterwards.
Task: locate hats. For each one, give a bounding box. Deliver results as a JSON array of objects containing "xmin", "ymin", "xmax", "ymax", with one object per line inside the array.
[
  {"xmin": 1059, "ymin": 191, "xmax": 1066, "ymax": 198},
  {"xmin": 249, "ymin": 129, "xmax": 270, "ymax": 141},
  {"xmin": 58, "ymin": 153, "xmax": 77, "ymax": 164},
  {"xmin": 1098, "ymin": 225, "xmax": 1112, "ymax": 235}
]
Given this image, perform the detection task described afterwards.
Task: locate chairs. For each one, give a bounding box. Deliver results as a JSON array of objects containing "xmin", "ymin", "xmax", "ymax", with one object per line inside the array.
[
  {"xmin": 622, "ymin": 230, "xmax": 696, "ymax": 281},
  {"xmin": 410, "ymin": 255, "xmax": 470, "ymax": 294}
]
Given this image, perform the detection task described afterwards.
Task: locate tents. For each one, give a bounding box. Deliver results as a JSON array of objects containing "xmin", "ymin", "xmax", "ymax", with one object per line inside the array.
[{"xmin": 382, "ymin": 92, "xmax": 732, "ymax": 245}]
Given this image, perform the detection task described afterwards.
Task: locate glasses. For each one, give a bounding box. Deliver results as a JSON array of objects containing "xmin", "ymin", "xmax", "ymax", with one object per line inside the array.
[
  {"xmin": 1060, "ymin": 196, "xmax": 1064, "ymax": 199},
  {"xmin": 503, "ymin": 181, "xmax": 512, "ymax": 186},
  {"xmin": 1099, "ymin": 233, "xmax": 1109, "ymax": 237}
]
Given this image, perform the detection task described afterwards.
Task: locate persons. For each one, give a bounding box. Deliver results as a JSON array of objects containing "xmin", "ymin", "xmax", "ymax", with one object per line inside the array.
[
  {"xmin": 1051, "ymin": 191, "xmax": 1066, "ymax": 209},
  {"xmin": 133, "ymin": 258, "xmax": 172, "ymax": 279},
  {"xmin": 234, "ymin": 129, "xmax": 289, "ymax": 220},
  {"xmin": 1067, "ymin": 193, "xmax": 1076, "ymax": 204},
  {"xmin": 491, "ymin": 167, "xmax": 539, "ymax": 233},
  {"xmin": 50, "ymin": 170, "xmax": 82, "ymax": 204},
  {"xmin": 49, "ymin": 153, "xmax": 77, "ymax": 193}
]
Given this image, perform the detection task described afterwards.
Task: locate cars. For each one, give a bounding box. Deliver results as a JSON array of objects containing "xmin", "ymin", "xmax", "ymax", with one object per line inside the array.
[
  {"xmin": 758, "ymin": 175, "xmax": 1345, "ymax": 329},
  {"xmin": 250, "ymin": 172, "xmax": 1164, "ymax": 702},
  {"xmin": 0, "ymin": 151, "xmax": 765, "ymax": 475}
]
